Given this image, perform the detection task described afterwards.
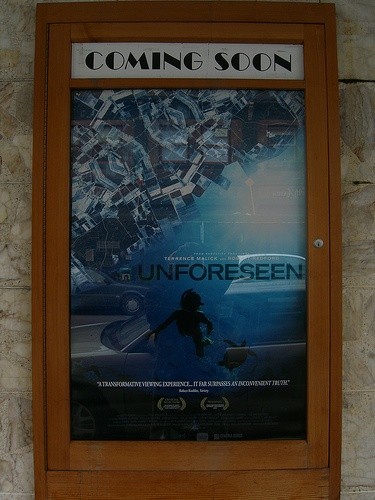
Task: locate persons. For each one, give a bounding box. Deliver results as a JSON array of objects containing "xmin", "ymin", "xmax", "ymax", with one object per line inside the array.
[{"xmin": 147, "ymin": 288, "xmax": 214, "ymax": 359}]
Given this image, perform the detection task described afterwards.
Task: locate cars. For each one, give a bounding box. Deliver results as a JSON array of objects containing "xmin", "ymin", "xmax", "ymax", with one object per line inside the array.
[
  {"xmin": 69, "ymin": 293, "xmax": 308, "ymax": 440},
  {"xmin": 224, "ymin": 253, "xmax": 307, "ymax": 294},
  {"xmin": 71, "ymin": 263, "xmax": 150, "ymax": 316}
]
